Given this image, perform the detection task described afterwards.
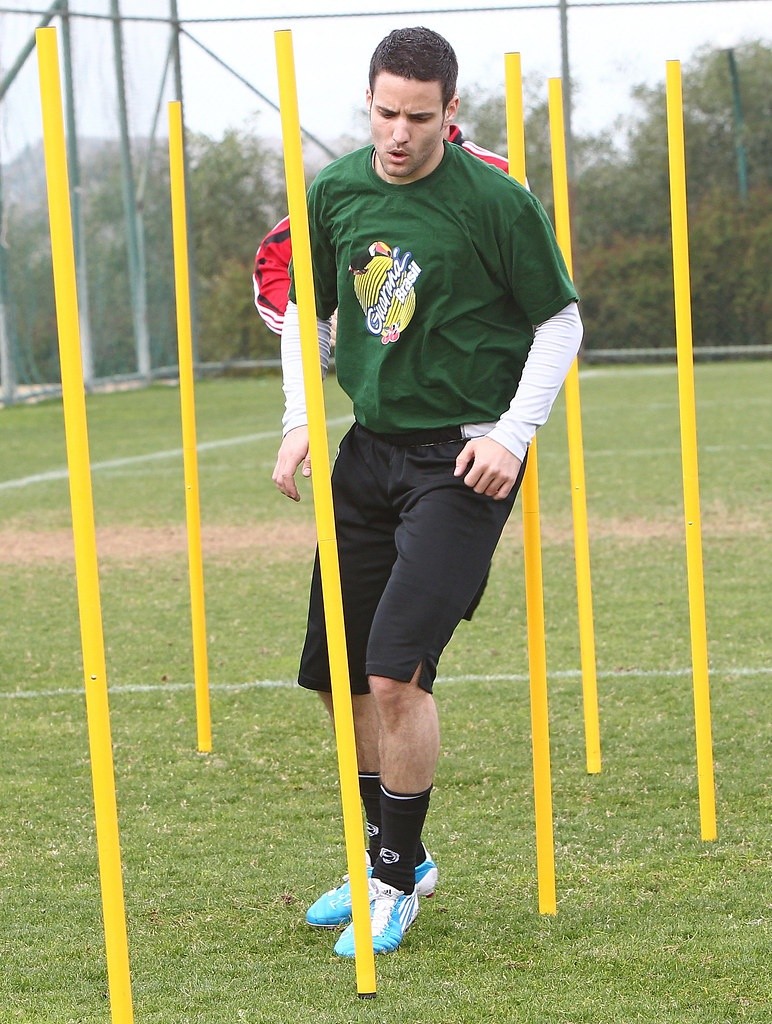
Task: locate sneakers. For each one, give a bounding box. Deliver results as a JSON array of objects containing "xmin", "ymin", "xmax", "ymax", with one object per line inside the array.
[
  {"xmin": 333, "ymin": 880, "xmax": 417, "ymax": 957},
  {"xmin": 308, "ymin": 842, "xmax": 438, "ymax": 926}
]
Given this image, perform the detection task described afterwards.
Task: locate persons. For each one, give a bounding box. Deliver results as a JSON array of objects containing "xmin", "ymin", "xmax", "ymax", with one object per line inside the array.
[{"xmin": 248, "ymin": 26, "xmax": 584, "ymax": 964}]
